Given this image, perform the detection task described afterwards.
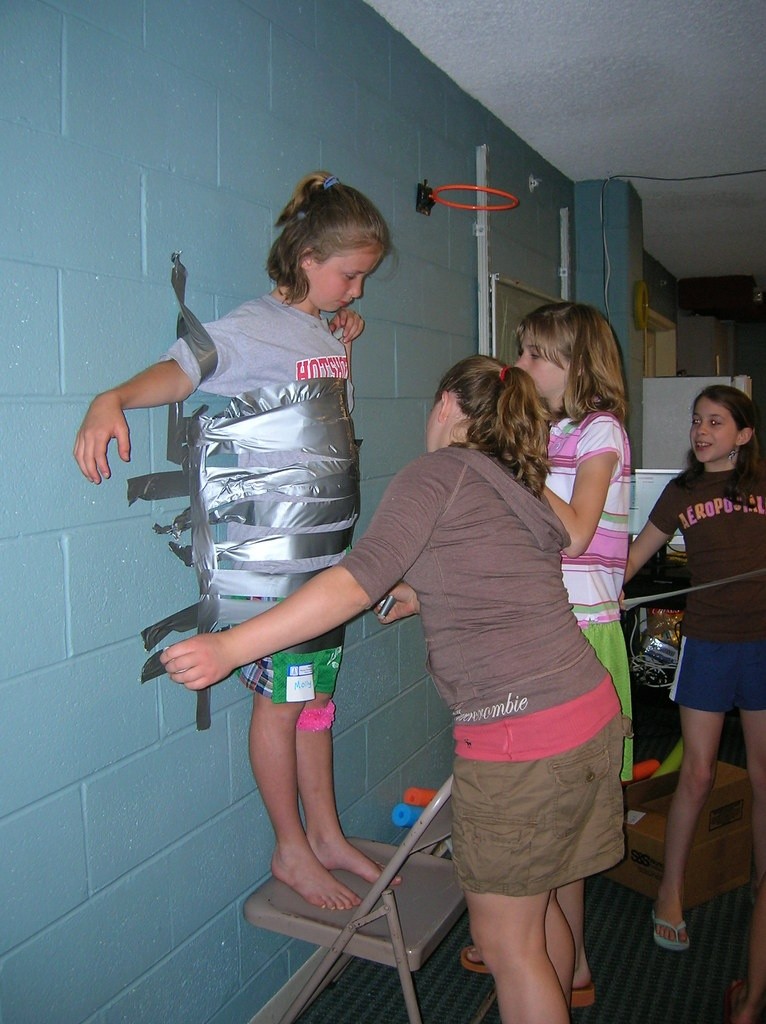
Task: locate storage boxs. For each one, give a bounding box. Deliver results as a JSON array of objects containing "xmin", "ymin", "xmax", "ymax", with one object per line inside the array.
[
  {"xmin": 599, "ymin": 758, "xmax": 754, "ymax": 911},
  {"xmin": 632, "ymin": 468, "xmax": 684, "ymax": 537}
]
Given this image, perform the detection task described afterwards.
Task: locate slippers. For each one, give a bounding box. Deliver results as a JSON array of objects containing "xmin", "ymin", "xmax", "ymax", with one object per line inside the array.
[
  {"xmin": 460, "ymin": 945, "xmax": 492, "ymax": 974},
  {"xmin": 571, "ymin": 983, "xmax": 595, "ymax": 1009},
  {"xmin": 651, "ymin": 909, "xmax": 689, "ymax": 951},
  {"xmin": 726, "ymin": 979, "xmax": 747, "ymax": 1024}
]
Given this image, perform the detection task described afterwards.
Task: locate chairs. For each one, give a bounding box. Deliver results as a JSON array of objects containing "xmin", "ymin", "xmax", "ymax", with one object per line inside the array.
[{"xmin": 242, "ymin": 775, "xmax": 498, "ymax": 1024}]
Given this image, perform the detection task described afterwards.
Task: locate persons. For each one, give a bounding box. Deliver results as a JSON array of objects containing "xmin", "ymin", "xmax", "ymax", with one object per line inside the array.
[
  {"xmin": 162, "ymin": 354, "xmax": 626, "ymax": 1024},
  {"xmin": 623, "ymin": 384, "xmax": 766, "ymax": 951},
  {"xmin": 459, "ymin": 302, "xmax": 635, "ymax": 1008},
  {"xmin": 72, "ymin": 163, "xmax": 390, "ymax": 913}
]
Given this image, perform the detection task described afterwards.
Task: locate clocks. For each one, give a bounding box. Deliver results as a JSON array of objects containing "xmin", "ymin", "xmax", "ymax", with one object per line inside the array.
[{"xmin": 634, "ymin": 280, "xmax": 650, "ymax": 330}]
